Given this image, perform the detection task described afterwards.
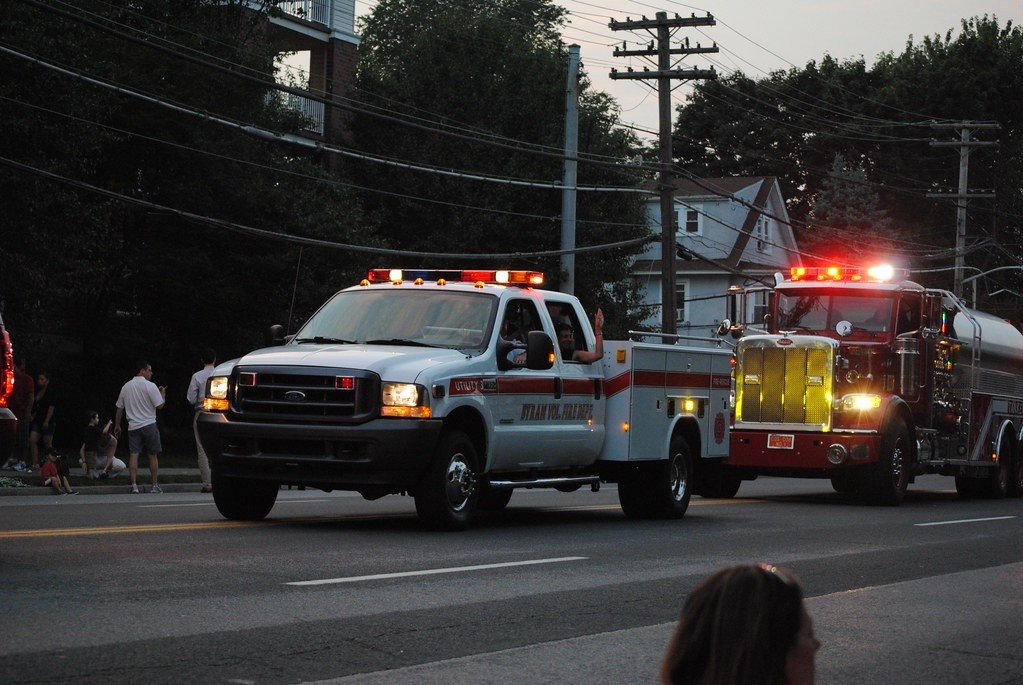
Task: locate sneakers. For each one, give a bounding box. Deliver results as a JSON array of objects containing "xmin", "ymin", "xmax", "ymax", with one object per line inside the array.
[
  {"xmin": 3, "ymin": 459, "xmax": 18, "ymax": 469},
  {"xmin": 11, "ymin": 462, "xmax": 26, "ymax": 472}
]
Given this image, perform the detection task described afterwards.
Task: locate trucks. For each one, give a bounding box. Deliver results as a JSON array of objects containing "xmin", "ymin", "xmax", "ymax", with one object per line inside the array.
[{"xmin": 196, "ymin": 268, "xmax": 733, "ymax": 535}]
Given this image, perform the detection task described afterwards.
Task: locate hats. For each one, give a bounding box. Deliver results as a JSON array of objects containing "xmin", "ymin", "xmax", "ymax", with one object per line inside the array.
[{"xmin": 44, "ymin": 447, "xmax": 58, "ymax": 456}]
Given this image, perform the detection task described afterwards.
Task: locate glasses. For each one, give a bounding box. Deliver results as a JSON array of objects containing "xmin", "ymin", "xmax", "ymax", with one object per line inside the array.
[{"xmin": 757, "ymin": 561, "xmax": 789, "ymax": 643}]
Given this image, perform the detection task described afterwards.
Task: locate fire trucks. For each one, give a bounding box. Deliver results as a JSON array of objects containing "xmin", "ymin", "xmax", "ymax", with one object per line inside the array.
[{"xmin": 716, "ymin": 265, "xmax": 1023, "ymax": 507}]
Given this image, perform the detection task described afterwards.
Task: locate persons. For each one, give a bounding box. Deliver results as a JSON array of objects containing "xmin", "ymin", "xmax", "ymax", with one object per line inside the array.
[
  {"xmin": 874, "ymin": 301, "xmax": 913, "ymax": 338},
  {"xmin": 0, "ymin": 359, "xmax": 126, "ymax": 496},
  {"xmin": 113, "ymin": 361, "xmax": 167, "ymax": 494},
  {"xmin": 554, "ymin": 307, "xmax": 605, "ymax": 363},
  {"xmin": 460, "ymin": 317, "xmax": 527, "ymax": 364},
  {"xmin": 187, "ymin": 349, "xmax": 217, "ymax": 493},
  {"xmin": 657, "ymin": 564, "xmax": 820, "ymax": 685}
]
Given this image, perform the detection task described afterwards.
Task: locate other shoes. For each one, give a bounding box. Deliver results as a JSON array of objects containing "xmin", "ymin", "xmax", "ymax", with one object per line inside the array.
[
  {"xmin": 130, "ymin": 485, "xmax": 139, "ymax": 494},
  {"xmin": 201, "ymin": 487, "xmax": 211, "ymax": 493},
  {"xmin": 67, "ymin": 490, "xmax": 79, "ymax": 495},
  {"xmin": 56, "ymin": 491, "xmax": 67, "ymax": 496},
  {"xmin": 151, "ymin": 485, "xmax": 163, "ymax": 493},
  {"xmin": 28, "ymin": 464, "xmax": 40, "ymax": 473}
]
